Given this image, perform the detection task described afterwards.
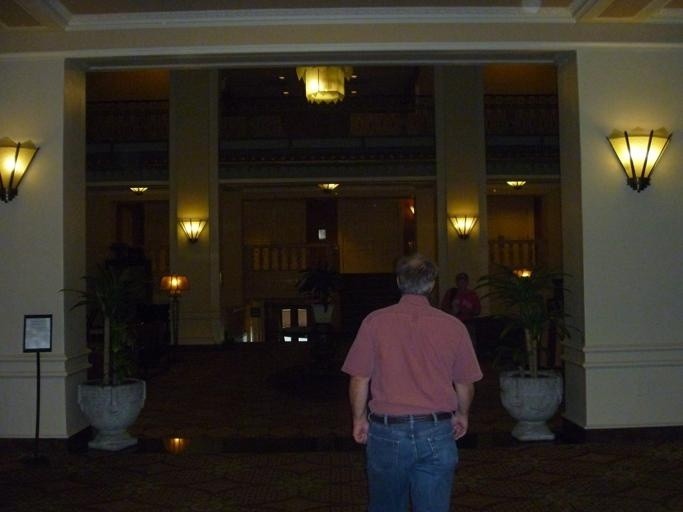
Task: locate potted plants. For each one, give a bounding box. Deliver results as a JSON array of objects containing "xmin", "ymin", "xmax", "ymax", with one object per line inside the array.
[
  {"xmin": 474, "ymin": 259, "xmax": 583, "ymax": 446},
  {"xmin": 57, "ymin": 252, "xmax": 166, "ymax": 454},
  {"xmin": 294, "ymin": 258, "xmax": 345, "ymax": 326}
]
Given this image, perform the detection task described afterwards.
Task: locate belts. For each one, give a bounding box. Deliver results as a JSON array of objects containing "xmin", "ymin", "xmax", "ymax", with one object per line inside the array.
[{"xmin": 369, "ymin": 412, "xmax": 457, "ymax": 425}]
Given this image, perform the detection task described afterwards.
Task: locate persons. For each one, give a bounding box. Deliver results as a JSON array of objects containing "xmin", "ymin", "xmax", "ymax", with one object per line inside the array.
[
  {"xmin": 340, "ymin": 248, "xmax": 484, "ymax": 511},
  {"xmin": 441, "ymin": 272, "xmax": 480, "ymax": 342}
]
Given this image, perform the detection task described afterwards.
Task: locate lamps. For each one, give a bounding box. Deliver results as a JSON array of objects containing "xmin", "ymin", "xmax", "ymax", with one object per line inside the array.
[
  {"xmin": 510, "ymin": 262, "xmax": 534, "ymax": 279},
  {"xmin": 318, "ymin": 182, "xmax": 340, "ymax": 193},
  {"xmin": 174, "ymin": 215, "xmax": 208, "ymax": 244},
  {"xmin": 447, "ymin": 212, "xmax": 481, "ymax": 241},
  {"xmin": 159, "ymin": 272, "xmax": 191, "ymax": 304},
  {"xmin": 606, "ymin": 123, "xmax": 675, "ymax": 195},
  {"xmin": 294, "ymin": 62, "xmax": 356, "ymax": 106},
  {"xmin": 127, "ymin": 186, "xmax": 151, "ymax": 195},
  {"xmin": 507, "ymin": 180, "xmax": 528, "ymax": 190},
  {"xmin": 0, "ymin": 134, "xmax": 43, "ymax": 206}
]
